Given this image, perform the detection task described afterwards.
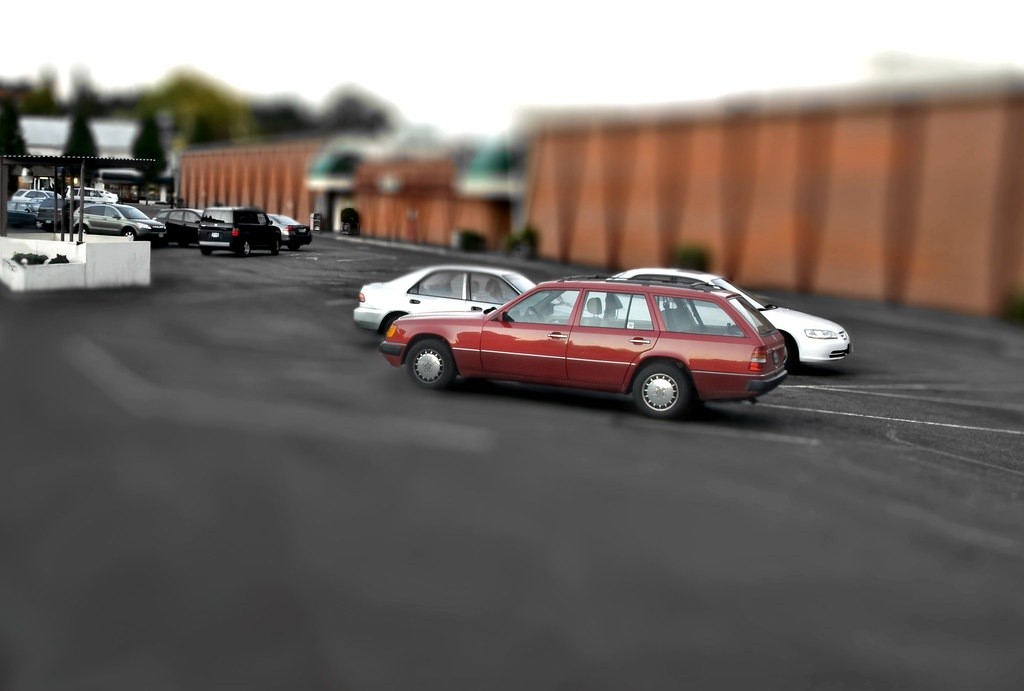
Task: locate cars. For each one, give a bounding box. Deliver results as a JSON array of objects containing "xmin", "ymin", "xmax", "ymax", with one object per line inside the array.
[
  {"xmin": 256, "ymin": 212, "xmax": 313, "ymax": 250},
  {"xmin": 7, "ymin": 184, "xmax": 120, "ymax": 229},
  {"xmin": 151, "ymin": 208, "xmax": 205, "ymax": 249},
  {"xmin": 70, "ymin": 203, "xmax": 168, "ymax": 246},
  {"xmin": 377, "ymin": 274, "xmax": 789, "ymax": 419},
  {"xmin": 535, "ymin": 266, "xmax": 854, "ymax": 369},
  {"xmin": 353, "ymin": 264, "xmax": 602, "ymax": 342}
]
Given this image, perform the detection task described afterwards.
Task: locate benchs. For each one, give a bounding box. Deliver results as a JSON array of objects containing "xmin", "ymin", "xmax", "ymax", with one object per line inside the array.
[
  {"xmin": 420, "ymin": 284, "xmax": 453, "ymax": 295},
  {"xmin": 662, "ymin": 307, "xmax": 695, "ymax": 333}
]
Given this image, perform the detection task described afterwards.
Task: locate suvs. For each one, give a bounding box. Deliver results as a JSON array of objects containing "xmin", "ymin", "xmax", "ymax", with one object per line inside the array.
[
  {"xmin": 197, "ymin": 206, "xmax": 283, "ymax": 257},
  {"xmin": 35, "ymin": 197, "xmax": 96, "ymax": 232}
]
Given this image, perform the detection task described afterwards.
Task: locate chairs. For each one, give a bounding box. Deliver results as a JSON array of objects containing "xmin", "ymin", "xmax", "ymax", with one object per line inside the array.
[
  {"xmin": 471, "ymin": 279, "xmax": 499, "ymax": 302},
  {"xmin": 583, "ymin": 295, "xmax": 623, "ymax": 326}
]
